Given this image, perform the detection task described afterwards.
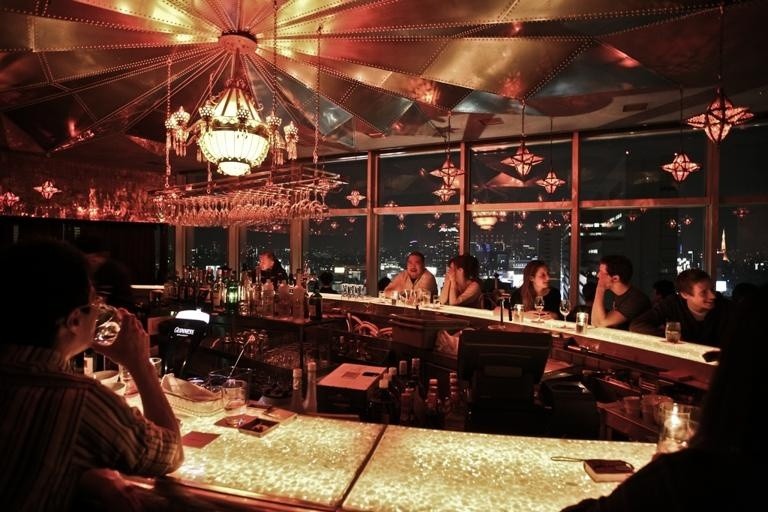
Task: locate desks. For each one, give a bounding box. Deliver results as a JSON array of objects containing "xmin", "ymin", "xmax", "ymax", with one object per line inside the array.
[{"xmin": 599, "ymin": 397, "xmax": 681, "ymax": 440}]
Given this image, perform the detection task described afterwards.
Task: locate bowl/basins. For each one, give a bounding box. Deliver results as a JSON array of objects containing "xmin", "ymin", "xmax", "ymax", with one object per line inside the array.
[
  {"xmin": 87, "ymin": 370, "xmax": 119, "ymax": 387},
  {"xmin": 101, "ymin": 382, "xmax": 125, "ymax": 397}
]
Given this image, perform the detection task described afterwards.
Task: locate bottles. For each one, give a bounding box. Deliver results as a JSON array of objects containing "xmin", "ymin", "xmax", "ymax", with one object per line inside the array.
[
  {"xmin": 174, "ymin": 265, "xmax": 236, "ymax": 317},
  {"xmin": 331, "ymin": 336, "xmax": 369, "ymax": 362},
  {"xmin": 237, "ymin": 270, "xmax": 251, "ymax": 316},
  {"xmin": 292, "ymin": 269, "xmax": 304, "ymax": 318},
  {"xmin": 261, "ymin": 278, "xmax": 274, "ymax": 314},
  {"xmin": 305, "ymin": 360, "xmax": 318, "ymax": 414},
  {"xmin": 274, "ymin": 289, "xmax": 280, "ymax": 314},
  {"xmin": 308, "ymin": 279, "xmax": 324, "ymax": 322},
  {"xmin": 320, "ymin": 357, "xmax": 330, "ymax": 375},
  {"xmin": 279, "ymin": 279, "xmax": 291, "ymax": 316},
  {"xmin": 251, "ymin": 266, "xmax": 264, "ymax": 314},
  {"xmin": 223, "ymin": 331, "xmax": 230, "ymax": 343},
  {"xmin": 288, "ymin": 367, "xmax": 306, "ymax": 415},
  {"xmin": 368, "ymin": 358, "xmax": 476, "ymax": 431},
  {"xmin": 234, "ymin": 333, "xmax": 241, "ymax": 343}
]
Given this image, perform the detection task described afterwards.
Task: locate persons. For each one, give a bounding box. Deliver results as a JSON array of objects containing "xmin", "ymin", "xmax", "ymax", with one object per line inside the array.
[
  {"xmin": 509, "ymin": 261, "xmax": 560, "ymax": 320},
  {"xmin": 257, "ymin": 250, "xmax": 287, "ymax": 291},
  {"xmin": 0, "ymin": 238, "xmax": 183, "ymax": 512},
  {"xmin": 565, "ymin": 256, "xmax": 767, "ymax": 355},
  {"xmin": 74, "ymin": 230, "xmax": 137, "ymax": 315},
  {"xmin": 319, "ymin": 272, "xmax": 337, "ymax": 294},
  {"xmin": 438, "ymin": 255, "xmax": 486, "ymax": 341},
  {"xmin": 561, "ymin": 314, "xmax": 767, "ymax": 512},
  {"xmin": 383, "ymin": 251, "xmax": 437, "ymax": 302}
]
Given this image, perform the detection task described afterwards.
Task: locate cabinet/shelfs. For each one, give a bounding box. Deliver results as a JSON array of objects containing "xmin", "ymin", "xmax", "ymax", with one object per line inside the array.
[{"xmin": 181, "ymin": 307, "xmax": 339, "ymax": 397}]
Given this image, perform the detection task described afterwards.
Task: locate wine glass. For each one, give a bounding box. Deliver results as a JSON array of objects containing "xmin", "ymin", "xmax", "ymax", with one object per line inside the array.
[
  {"xmin": 532, "ymin": 296, "xmax": 545, "ymax": 325},
  {"xmin": 558, "ymin": 299, "xmax": 572, "ymax": 329},
  {"xmin": 155, "ymin": 177, "xmax": 330, "ymax": 227}
]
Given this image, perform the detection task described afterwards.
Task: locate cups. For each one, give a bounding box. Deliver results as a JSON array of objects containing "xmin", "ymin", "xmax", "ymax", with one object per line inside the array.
[
  {"xmin": 379, "ymin": 290, "xmax": 385, "ymax": 302},
  {"xmin": 149, "ymin": 357, "xmax": 162, "ymax": 379},
  {"xmin": 514, "ymin": 304, "xmax": 525, "ymax": 322},
  {"xmin": 659, "ymin": 402, "xmax": 693, "ymax": 451},
  {"xmin": 341, "ymin": 283, "xmax": 365, "ymax": 302},
  {"xmin": 575, "ymin": 311, "xmax": 589, "ymax": 333},
  {"xmin": 433, "ymin": 294, "xmax": 441, "ymax": 308},
  {"xmin": 624, "ymin": 396, "xmax": 640, "ymax": 418},
  {"xmin": 405, "ymin": 289, "xmax": 413, "ymax": 304},
  {"xmin": 665, "ymin": 321, "xmax": 681, "ymax": 344},
  {"xmin": 423, "ymin": 290, "xmax": 431, "ymax": 308},
  {"xmin": 655, "ymin": 396, "xmax": 673, "ymax": 426},
  {"xmin": 641, "ymin": 398, "xmax": 655, "ymax": 426},
  {"xmin": 221, "ymin": 378, "xmax": 248, "ymax": 412},
  {"xmin": 95, "ymin": 306, "xmax": 121, "ymax": 347},
  {"xmin": 391, "ymin": 290, "xmax": 397, "ymax": 305},
  {"xmin": 118, "ymin": 364, "xmax": 138, "ymax": 394}
]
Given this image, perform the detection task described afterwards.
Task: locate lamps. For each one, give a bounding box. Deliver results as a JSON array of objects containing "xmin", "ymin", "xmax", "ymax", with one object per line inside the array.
[
  {"xmin": 30, "ymin": 180, "xmax": 63, "ymax": 203},
  {"xmin": 663, "ymin": 90, "xmax": 701, "ymax": 183},
  {"xmin": 688, "ymin": 77, "xmax": 753, "ymax": 143},
  {"xmin": 0, "ymin": 191, "xmax": 21, "ymax": 209},
  {"xmin": 161, "ymin": 33, "xmax": 301, "ymax": 178},
  {"xmin": 428, "ymin": 96, "xmax": 569, "ymax": 203},
  {"xmin": 348, "ymin": 152, "xmax": 365, "ymax": 206}
]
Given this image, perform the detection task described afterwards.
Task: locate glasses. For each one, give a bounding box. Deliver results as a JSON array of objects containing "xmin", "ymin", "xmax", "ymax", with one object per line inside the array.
[{"xmin": 79, "ymin": 296, "xmax": 103, "ymax": 312}]
